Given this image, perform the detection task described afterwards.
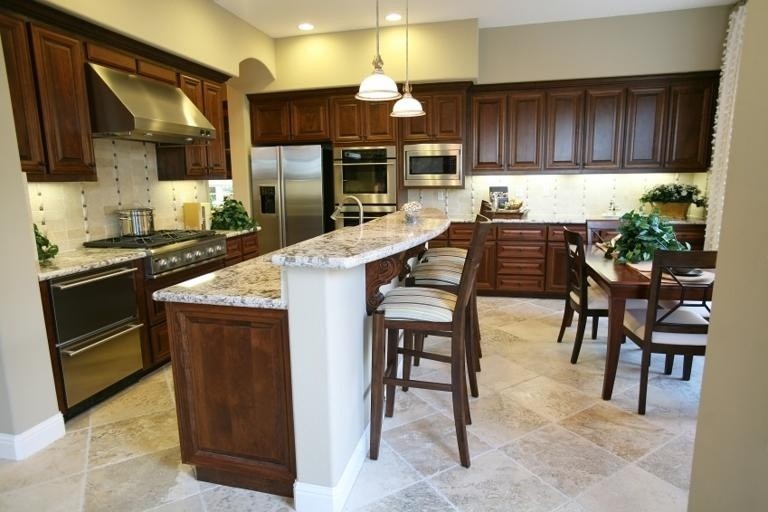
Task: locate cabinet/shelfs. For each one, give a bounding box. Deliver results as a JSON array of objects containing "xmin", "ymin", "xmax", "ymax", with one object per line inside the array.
[
  {"xmin": 622, "ymin": 69, "xmax": 719, "ymax": 175},
  {"xmin": 467, "ymin": 81, "xmax": 545, "ymax": 174},
  {"xmin": 399, "ymin": 80, "xmax": 467, "ymax": 143},
  {"xmin": 144, "ymin": 260, "xmax": 223, "ymax": 366},
  {"xmin": 0, "ymin": 11, "xmax": 98, "ymax": 183},
  {"xmin": 329, "ymin": 88, "xmax": 398, "ymax": 144},
  {"xmin": 426, "ymin": 224, "xmax": 496, "ymax": 298},
  {"xmin": 497, "ymin": 221, "xmax": 550, "ymax": 298},
  {"xmin": 544, "ymin": 223, "xmax": 627, "ymax": 300},
  {"xmin": 251, "ymin": 90, "xmax": 329, "ymax": 144},
  {"xmin": 669, "ymin": 222, "xmax": 708, "ymax": 249},
  {"xmin": 225, "ymin": 235, "xmax": 261, "ymax": 266},
  {"xmin": 156, "ymin": 71, "xmax": 227, "ymax": 181},
  {"xmin": 546, "ymin": 77, "xmax": 626, "ymax": 175}
]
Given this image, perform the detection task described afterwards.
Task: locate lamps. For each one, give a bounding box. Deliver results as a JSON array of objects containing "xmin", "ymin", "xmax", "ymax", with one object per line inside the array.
[
  {"xmin": 354, "ymin": 0, "xmax": 402, "ymax": 102},
  {"xmin": 389, "ymin": 0, "xmax": 427, "ymax": 118}
]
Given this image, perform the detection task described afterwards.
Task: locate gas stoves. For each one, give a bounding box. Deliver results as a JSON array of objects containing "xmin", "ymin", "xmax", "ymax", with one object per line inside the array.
[{"xmin": 84, "ymin": 229, "xmax": 216, "ymax": 248}]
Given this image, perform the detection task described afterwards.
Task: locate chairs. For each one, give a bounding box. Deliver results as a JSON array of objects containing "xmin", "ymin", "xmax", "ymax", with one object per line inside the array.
[
  {"xmin": 622, "ymin": 250, "xmax": 717, "ymax": 415},
  {"xmin": 405, "ymin": 209, "xmax": 481, "ymax": 398},
  {"xmin": 420, "ymin": 200, "xmax": 495, "ymax": 357},
  {"xmin": 557, "ymin": 226, "xmax": 626, "ymax": 365},
  {"xmin": 368, "ymin": 213, "xmax": 494, "ymax": 469}
]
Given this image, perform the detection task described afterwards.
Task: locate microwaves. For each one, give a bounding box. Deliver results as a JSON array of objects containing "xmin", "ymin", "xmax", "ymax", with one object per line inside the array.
[{"xmin": 403, "ymin": 143, "xmax": 464, "ymax": 188}]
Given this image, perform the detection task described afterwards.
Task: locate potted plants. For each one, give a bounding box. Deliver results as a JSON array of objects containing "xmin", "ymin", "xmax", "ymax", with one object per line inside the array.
[
  {"xmin": 638, "ymin": 182, "xmax": 700, "ymax": 221},
  {"xmin": 686, "ymin": 195, "xmax": 708, "ymax": 221}
]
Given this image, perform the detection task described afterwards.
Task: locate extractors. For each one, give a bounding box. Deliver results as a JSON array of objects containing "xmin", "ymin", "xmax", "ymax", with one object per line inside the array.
[{"xmin": 88, "ymin": 61, "xmax": 216, "ymax": 146}]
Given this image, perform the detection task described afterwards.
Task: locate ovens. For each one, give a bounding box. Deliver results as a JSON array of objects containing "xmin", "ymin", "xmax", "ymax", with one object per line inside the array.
[
  {"xmin": 332, "ymin": 205, "xmax": 396, "ymax": 230},
  {"xmin": 332, "ymin": 146, "xmax": 397, "ymax": 206}
]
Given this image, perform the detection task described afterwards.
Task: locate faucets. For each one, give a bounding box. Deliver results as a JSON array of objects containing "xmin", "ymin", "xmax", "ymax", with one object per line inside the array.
[{"xmin": 330, "ymin": 196, "xmax": 363, "ymax": 225}]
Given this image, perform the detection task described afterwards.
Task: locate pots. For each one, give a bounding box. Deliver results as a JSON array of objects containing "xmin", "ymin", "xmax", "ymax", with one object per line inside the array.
[{"xmin": 115, "ymin": 209, "xmax": 153, "ymax": 236}]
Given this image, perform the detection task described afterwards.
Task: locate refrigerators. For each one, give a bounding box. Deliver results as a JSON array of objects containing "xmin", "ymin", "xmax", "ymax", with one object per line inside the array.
[{"xmin": 251, "ymin": 144, "xmax": 332, "ymax": 253}]
